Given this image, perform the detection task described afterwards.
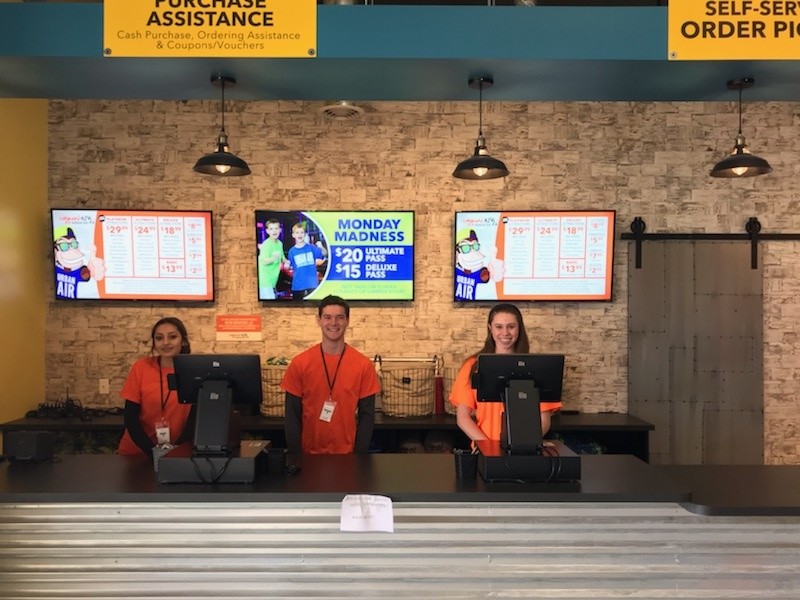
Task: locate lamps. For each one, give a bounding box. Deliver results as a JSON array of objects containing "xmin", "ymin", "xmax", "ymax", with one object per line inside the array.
[
  {"xmin": 453, "ymin": 78, "xmax": 510, "ymax": 180},
  {"xmin": 193, "ymin": 72, "xmax": 252, "ymax": 176},
  {"xmin": 709, "ymin": 77, "xmax": 773, "ymax": 178}
]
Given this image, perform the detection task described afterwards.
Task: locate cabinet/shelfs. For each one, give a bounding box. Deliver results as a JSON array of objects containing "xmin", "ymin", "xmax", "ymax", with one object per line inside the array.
[{"xmin": 0, "ymin": 416, "xmax": 656, "ymax": 466}]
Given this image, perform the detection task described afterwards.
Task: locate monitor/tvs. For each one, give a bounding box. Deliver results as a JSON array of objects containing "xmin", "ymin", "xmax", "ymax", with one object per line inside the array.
[
  {"xmin": 477, "ymin": 352, "xmax": 564, "ymax": 402},
  {"xmin": 256, "ymin": 209, "xmax": 414, "ymax": 302},
  {"xmin": 453, "ymin": 210, "xmax": 616, "ymax": 302},
  {"xmin": 50, "ymin": 207, "xmax": 215, "ymax": 303},
  {"xmin": 172, "ymin": 354, "xmax": 262, "ymax": 403}
]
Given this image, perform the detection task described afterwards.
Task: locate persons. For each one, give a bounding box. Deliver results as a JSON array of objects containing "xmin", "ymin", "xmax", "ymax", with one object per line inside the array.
[
  {"xmin": 117, "ymin": 318, "xmax": 193, "ymax": 459},
  {"xmin": 284, "ymin": 223, "xmax": 326, "ymax": 299},
  {"xmin": 448, "ymin": 304, "xmax": 562, "ymax": 451},
  {"xmin": 280, "ymin": 294, "xmax": 381, "ymax": 455},
  {"xmin": 258, "ymin": 217, "xmax": 290, "ymax": 299}
]
